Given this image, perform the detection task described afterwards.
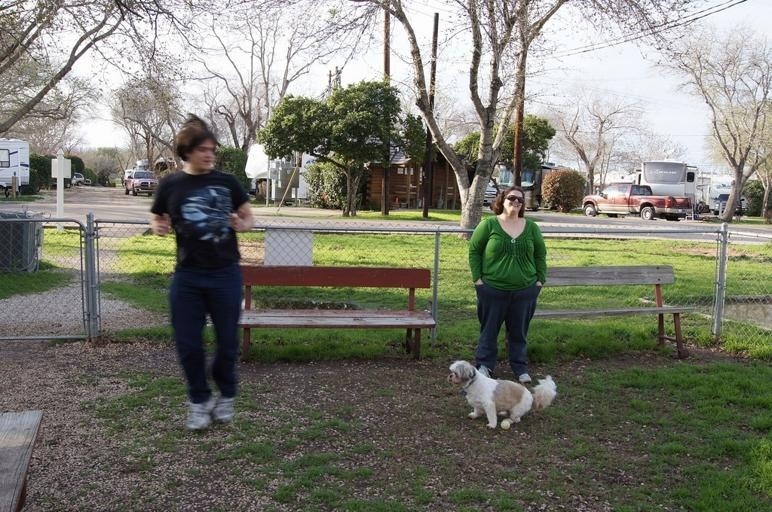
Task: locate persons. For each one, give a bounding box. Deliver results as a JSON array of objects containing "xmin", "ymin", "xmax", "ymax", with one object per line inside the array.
[
  {"xmin": 147, "ymin": 111, "xmax": 258, "ymax": 433},
  {"xmin": 466, "ymin": 185, "xmax": 547, "ymax": 385}
]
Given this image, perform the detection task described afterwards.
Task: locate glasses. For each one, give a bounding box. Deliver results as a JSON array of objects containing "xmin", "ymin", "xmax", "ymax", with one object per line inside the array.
[{"xmin": 503, "ymin": 194, "xmax": 525, "ymax": 204}]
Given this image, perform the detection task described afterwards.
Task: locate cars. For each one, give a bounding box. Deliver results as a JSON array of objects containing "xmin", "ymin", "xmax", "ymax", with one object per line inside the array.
[
  {"xmin": 69, "ymin": 171, "xmax": 86, "ymax": 187},
  {"xmin": 124, "ymin": 171, "xmax": 160, "ymax": 197}
]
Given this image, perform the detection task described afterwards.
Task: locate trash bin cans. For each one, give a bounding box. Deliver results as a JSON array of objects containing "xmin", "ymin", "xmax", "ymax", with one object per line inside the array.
[{"xmin": 0, "ymin": 210, "xmax": 44, "ymax": 272}]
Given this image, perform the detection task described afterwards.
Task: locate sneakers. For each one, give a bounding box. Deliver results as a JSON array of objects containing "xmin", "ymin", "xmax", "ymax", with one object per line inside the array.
[
  {"xmin": 212, "ymin": 395, "xmax": 235, "ymax": 423},
  {"xmin": 477, "ymin": 365, "xmax": 492, "ymax": 377},
  {"xmin": 186, "ymin": 396, "xmax": 217, "ymax": 432},
  {"xmin": 513, "ymin": 373, "xmax": 531, "ymax": 384}
]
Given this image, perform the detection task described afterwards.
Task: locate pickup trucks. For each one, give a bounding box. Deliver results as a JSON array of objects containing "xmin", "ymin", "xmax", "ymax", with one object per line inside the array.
[{"xmin": 580, "ymin": 182, "xmax": 693, "ymax": 221}]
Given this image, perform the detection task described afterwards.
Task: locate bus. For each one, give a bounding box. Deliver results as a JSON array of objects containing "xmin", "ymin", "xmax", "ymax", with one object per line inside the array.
[{"xmin": 497, "ymin": 160, "xmax": 587, "ymax": 212}]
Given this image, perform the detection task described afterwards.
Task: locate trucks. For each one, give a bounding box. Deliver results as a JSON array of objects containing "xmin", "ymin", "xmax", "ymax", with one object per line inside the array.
[
  {"xmin": 0, "ymin": 137, "xmax": 30, "ymax": 198},
  {"xmin": 634, "ymin": 160, "xmax": 752, "ymax": 221}
]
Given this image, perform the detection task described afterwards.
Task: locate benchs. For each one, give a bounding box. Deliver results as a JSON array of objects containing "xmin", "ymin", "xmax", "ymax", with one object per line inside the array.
[
  {"xmin": 530, "ymin": 265, "xmax": 695, "ymax": 359},
  {"xmin": 208, "ymin": 263, "xmax": 437, "ymax": 361}
]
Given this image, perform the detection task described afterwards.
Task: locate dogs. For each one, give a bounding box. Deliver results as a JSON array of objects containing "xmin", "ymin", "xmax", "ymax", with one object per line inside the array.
[{"xmin": 447, "ymin": 359, "xmax": 558, "ymax": 430}]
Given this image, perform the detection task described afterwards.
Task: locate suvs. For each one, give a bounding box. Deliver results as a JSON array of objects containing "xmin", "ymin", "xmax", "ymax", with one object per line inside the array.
[{"xmin": 482, "ymin": 181, "xmax": 500, "ymax": 210}]
